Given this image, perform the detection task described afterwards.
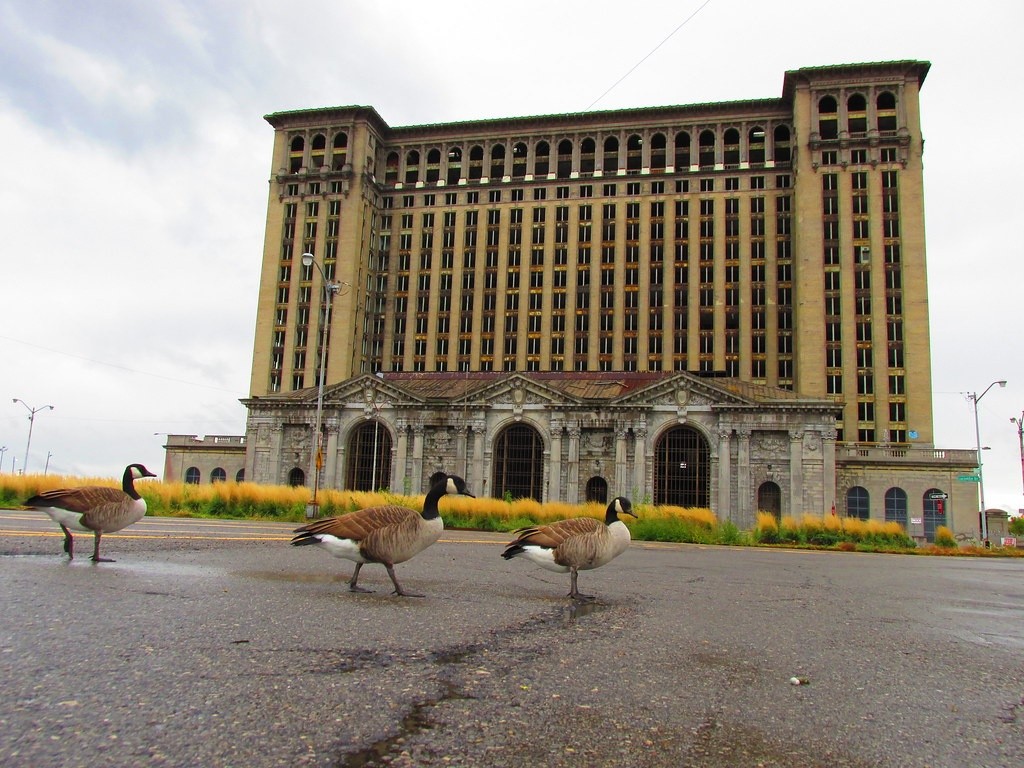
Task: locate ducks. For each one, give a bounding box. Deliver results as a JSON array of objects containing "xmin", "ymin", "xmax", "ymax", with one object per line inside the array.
[
  {"xmin": 23, "ymin": 464, "xmax": 157, "ymax": 562},
  {"xmin": 290, "ymin": 475, "xmax": 476, "ymax": 597},
  {"xmin": 500, "ymin": 496, "xmax": 638, "ymax": 602}
]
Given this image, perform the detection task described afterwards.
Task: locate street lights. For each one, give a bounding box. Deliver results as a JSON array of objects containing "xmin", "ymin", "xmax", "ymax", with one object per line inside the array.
[
  {"xmin": 12, "ymin": 398, "xmax": 54, "ymax": 474},
  {"xmin": 301, "ymin": 252, "xmax": 341, "ymax": 517},
  {"xmin": 968, "ymin": 380, "xmax": 1008, "ymax": 538},
  {"xmin": 1009, "ymin": 416, "xmax": 1024, "ymax": 492},
  {"xmin": 0, "ymin": 446, "xmax": 8, "ymax": 471}
]
